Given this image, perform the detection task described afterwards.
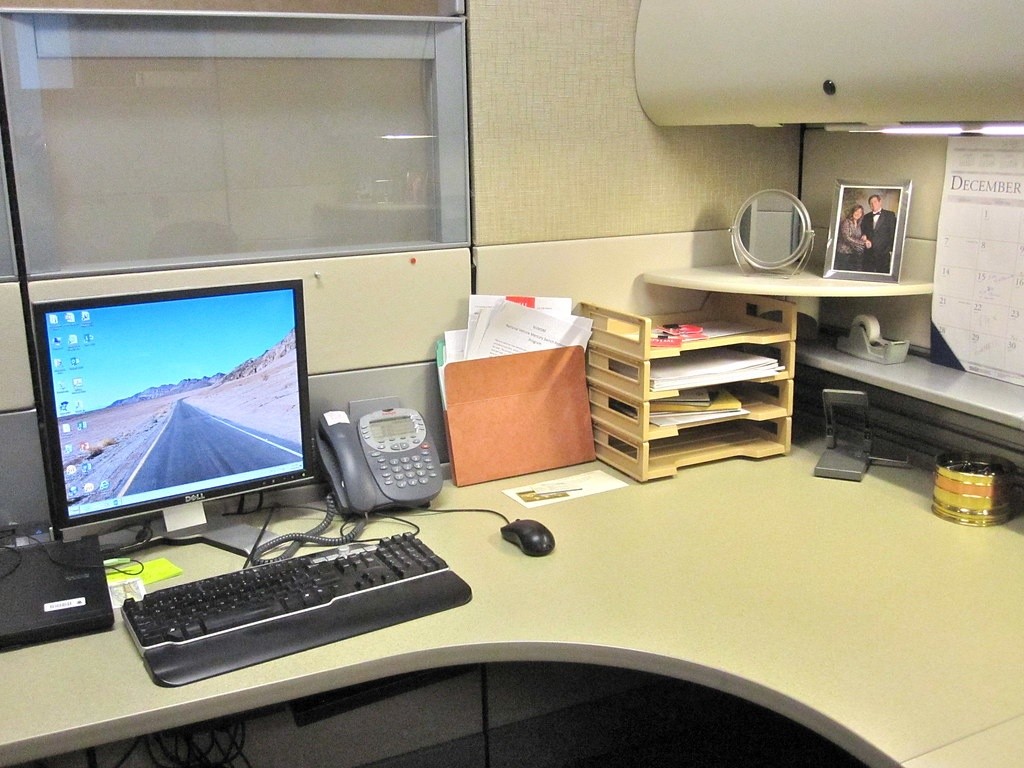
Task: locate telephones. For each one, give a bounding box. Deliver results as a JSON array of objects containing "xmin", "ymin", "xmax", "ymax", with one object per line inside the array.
[{"xmin": 313, "ymin": 394, "xmax": 443, "ymax": 520}]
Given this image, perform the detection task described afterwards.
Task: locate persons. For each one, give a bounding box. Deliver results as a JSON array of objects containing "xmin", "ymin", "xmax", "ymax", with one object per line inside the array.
[{"xmin": 837, "ymin": 194, "xmax": 896, "ymax": 273}]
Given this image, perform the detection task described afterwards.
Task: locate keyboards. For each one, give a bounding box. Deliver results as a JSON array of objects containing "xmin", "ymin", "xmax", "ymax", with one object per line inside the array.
[{"xmin": 121, "ymin": 531, "xmax": 471, "ymax": 687}]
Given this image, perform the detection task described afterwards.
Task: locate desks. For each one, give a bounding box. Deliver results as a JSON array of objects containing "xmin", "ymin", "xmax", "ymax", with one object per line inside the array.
[{"xmin": 0, "ymin": 419, "xmax": 1024, "ymax": 768}]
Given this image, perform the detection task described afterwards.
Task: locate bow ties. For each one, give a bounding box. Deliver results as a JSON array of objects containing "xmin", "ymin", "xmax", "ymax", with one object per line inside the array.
[{"xmin": 873, "ymin": 212, "xmax": 880, "ymax": 217}]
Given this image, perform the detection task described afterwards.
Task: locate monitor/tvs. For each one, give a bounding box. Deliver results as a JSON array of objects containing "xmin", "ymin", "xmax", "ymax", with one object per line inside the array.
[{"xmin": 31, "ymin": 279, "xmax": 315, "ymax": 600}]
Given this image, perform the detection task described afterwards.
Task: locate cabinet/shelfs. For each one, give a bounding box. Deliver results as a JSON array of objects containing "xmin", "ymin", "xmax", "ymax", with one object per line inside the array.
[{"xmin": 582, "ymin": 297, "xmax": 800, "ymax": 481}]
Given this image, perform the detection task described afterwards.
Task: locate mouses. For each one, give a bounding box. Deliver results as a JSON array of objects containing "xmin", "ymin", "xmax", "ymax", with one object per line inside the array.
[{"xmin": 500, "ymin": 518, "xmax": 555, "ymax": 557}]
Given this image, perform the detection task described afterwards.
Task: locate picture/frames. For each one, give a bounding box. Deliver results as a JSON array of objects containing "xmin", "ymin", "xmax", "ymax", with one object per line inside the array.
[{"xmin": 822, "ymin": 178, "xmax": 912, "ymax": 284}]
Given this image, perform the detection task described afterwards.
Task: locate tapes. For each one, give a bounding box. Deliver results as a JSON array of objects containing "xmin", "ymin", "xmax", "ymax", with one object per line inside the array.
[{"xmin": 852, "ymin": 312, "xmax": 880, "ymax": 345}]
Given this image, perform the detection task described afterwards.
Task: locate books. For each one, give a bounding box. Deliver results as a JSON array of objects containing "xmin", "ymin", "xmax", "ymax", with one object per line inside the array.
[
  {"xmin": 608, "ymin": 347, "xmax": 786, "ymax": 427},
  {"xmin": 435, "ymin": 295, "xmax": 596, "ymax": 488}
]
